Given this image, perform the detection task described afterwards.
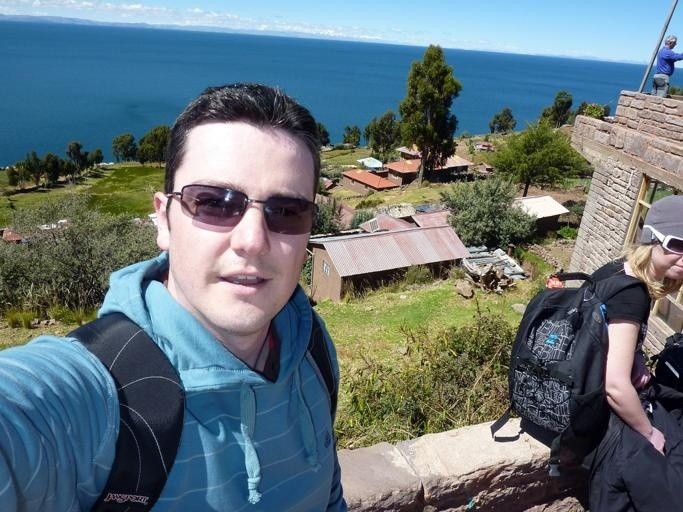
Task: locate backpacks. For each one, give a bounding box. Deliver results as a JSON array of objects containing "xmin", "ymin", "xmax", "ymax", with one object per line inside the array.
[
  {"xmin": 509, "ymin": 271, "xmax": 650, "ymax": 478},
  {"xmin": 654, "ymin": 333, "xmax": 681, "ymax": 387}
]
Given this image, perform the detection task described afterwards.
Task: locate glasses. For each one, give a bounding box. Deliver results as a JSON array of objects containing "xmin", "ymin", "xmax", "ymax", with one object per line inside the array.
[
  {"xmin": 168, "ymin": 184, "xmax": 318, "ymax": 235},
  {"xmin": 643, "ymin": 224, "xmax": 683, "ymax": 255}
]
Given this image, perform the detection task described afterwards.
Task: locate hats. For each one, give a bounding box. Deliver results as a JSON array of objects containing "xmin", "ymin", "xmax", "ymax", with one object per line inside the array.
[{"xmin": 639, "ymin": 194, "xmax": 683, "ymax": 245}]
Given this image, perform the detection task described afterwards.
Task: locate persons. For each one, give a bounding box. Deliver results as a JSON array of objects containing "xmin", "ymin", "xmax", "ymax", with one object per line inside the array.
[
  {"xmin": 578, "ymin": 195, "xmax": 683, "ymax": 512},
  {"xmin": 0, "ymin": 84, "xmax": 348, "ymax": 512},
  {"xmin": 651, "ymin": 34, "xmax": 683, "ymax": 98}
]
